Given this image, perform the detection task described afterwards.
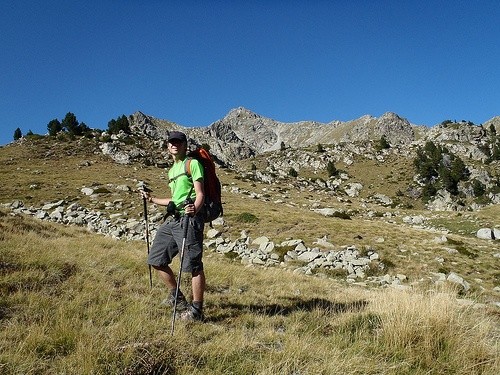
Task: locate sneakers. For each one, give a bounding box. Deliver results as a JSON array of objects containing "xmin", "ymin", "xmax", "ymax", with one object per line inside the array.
[
  {"xmin": 180, "ymin": 306, "xmax": 204, "ymax": 325},
  {"xmin": 155, "ymin": 295, "xmax": 187, "ymax": 312}
]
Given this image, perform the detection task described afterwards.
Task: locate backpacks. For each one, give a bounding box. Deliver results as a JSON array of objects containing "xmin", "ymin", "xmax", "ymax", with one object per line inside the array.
[{"xmin": 186, "ymin": 148, "xmax": 222, "ymax": 223}]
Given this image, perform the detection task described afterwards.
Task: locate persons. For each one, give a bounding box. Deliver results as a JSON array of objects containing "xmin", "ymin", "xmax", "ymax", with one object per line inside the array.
[{"xmin": 140, "ymin": 131, "xmax": 205, "ymax": 324}]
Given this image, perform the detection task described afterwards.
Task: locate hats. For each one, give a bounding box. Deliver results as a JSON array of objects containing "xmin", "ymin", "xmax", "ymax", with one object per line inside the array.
[{"xmin": 168, "ymin": 132, "xmax": 187, "ymax": 143}]
